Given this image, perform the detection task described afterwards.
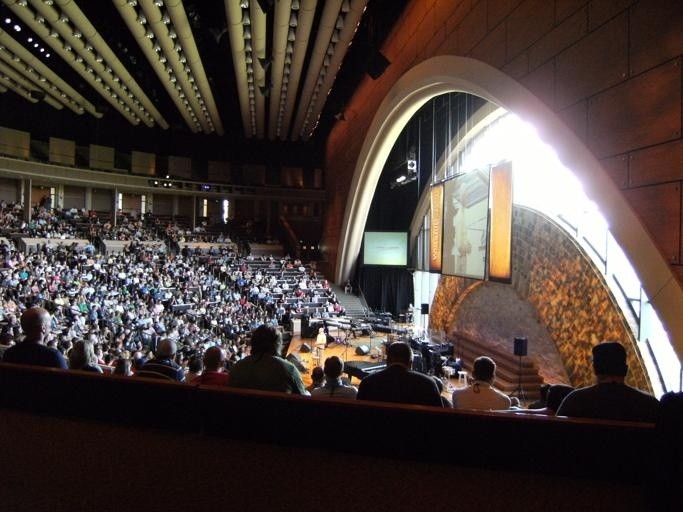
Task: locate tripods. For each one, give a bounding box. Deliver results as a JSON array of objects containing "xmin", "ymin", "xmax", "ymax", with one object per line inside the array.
[
  {"xmin": 508, "ymin": 356, "xmax": 528, "ymax": 405},
  {"xmin": 420, "ymin": 315, "xmax": 429, "ymax": 340}
]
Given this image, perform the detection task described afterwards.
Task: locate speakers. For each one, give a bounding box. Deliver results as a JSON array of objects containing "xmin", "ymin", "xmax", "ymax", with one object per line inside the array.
[
  {"xmin": 355, "ymin": 344, "xmax": 370, "ymax": 355},
  {"xmin": 287, "ymin": 352, "xmax": 306, "ymax": 372},
  {"xmin": 300, "ymin": 343, "xmax": 311, "ymax": 353},
  {"xmin": 514, "ymin": 337, "xmax": 528, "ymax": 356},
  {"xmin": 421, "ymin": 304, "xmax": 429, "ymax": 314}
]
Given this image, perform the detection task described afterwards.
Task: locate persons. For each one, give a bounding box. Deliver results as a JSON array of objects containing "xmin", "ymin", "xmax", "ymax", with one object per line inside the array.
[
  {"xmin": 452, "ymin": 356, "xmax": 511, "ymax": 411},
  {"xmin": 355, "ymin": 342, "xmax": 444, "ymax": 407},
  {"xmin": 406, "ymin": 302, "xmax": 414, "ymax": 322},
  {"xmin": 554, "ymin": 343, "xmax": 660, "ymax": 423},
  {"xmin": 528, "ymin": 383, "xmax": 550, "ymax": 409},
  {"xmin": 431, "ymin": 375, "xmax": 453, "ymax": 409},
  {"xmin": 1, "ymin": 197, "xmax": 359, "ymax": 402}
]
